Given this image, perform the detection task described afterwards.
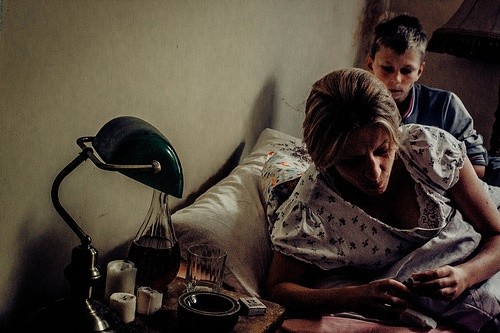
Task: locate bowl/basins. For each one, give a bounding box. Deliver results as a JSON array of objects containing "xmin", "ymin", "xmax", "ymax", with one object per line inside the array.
[{"xmin": 177, "ymin": 291, "xmax": 241, "ymax": 333}]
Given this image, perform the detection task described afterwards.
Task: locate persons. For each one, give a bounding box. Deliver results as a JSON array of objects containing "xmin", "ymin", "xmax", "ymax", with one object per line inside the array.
[
  {"xmin": 264, "ymin": 68, "xmax": 500, "ymax": 320},
  {"xmin": 363, "ymin": 15, "xmax": 500, "ymax": 187}
]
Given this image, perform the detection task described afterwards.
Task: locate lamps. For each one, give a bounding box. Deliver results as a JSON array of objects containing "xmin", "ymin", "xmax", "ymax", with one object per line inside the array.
[
  {"xmin": 425, "ymin": 0, "xmax": 500, "ymax": 187},
  {"xmin": 50, "ymin": 116, "xmax": 183, "ymax": 333}
]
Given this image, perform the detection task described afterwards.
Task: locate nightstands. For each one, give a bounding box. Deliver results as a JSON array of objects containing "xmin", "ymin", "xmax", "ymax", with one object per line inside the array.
[{"xmin": 168, "ymin": 275, "xmax": 285, "ymax": 333}]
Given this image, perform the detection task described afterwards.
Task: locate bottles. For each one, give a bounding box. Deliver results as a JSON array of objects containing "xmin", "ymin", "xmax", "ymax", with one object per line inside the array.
[{"xmin": 128, "ymin": 188, "xmax": 182, "ymax": 292}]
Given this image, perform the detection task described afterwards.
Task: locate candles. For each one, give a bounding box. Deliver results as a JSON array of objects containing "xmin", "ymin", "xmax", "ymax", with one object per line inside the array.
[{"xmin": 104, "ymin": 259, "xmax": 163, "ymax": 323}]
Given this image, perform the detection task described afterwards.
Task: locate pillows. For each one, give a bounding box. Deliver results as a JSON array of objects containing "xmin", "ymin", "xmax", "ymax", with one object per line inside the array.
[{"xmin": 169, "ymin": 128, "xmax": 313, "ymax": 298}]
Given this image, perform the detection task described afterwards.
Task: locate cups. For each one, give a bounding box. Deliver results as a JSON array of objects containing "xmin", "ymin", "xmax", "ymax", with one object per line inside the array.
[{"xmin": 186, "ymin": 243, "xmax": 228, "ymax": 295}]
